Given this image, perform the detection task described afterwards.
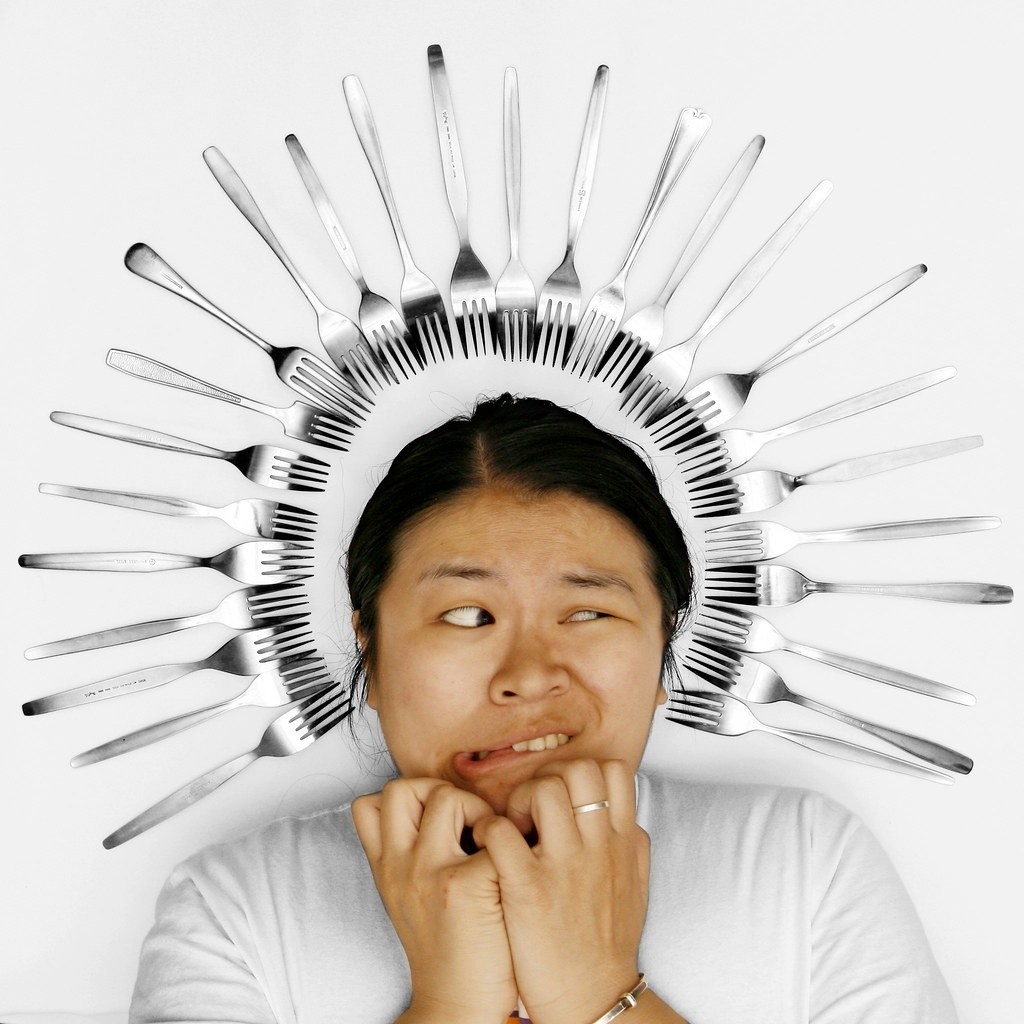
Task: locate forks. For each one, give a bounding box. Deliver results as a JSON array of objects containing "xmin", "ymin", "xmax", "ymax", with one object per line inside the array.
[{"xmin": 15, "ymin": 42, "xmax": 1012, "ymax": 850}]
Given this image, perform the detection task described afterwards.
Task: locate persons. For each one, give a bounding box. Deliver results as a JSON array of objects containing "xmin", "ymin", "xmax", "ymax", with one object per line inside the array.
[{"xmin": 125, "ymin": 392, "xmax": 960, "ymax": 1024}]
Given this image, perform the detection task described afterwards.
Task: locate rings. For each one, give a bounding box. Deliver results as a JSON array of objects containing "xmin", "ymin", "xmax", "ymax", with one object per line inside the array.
[{"xmin": 573, "ymin": 800, "xmax": 609, "ymax": 814}]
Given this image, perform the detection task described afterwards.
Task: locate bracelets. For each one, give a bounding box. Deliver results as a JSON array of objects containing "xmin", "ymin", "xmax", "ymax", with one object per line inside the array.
[{"xmin": 591, "ymin": 973, "xmax": 648, "ymax": 1024}]
